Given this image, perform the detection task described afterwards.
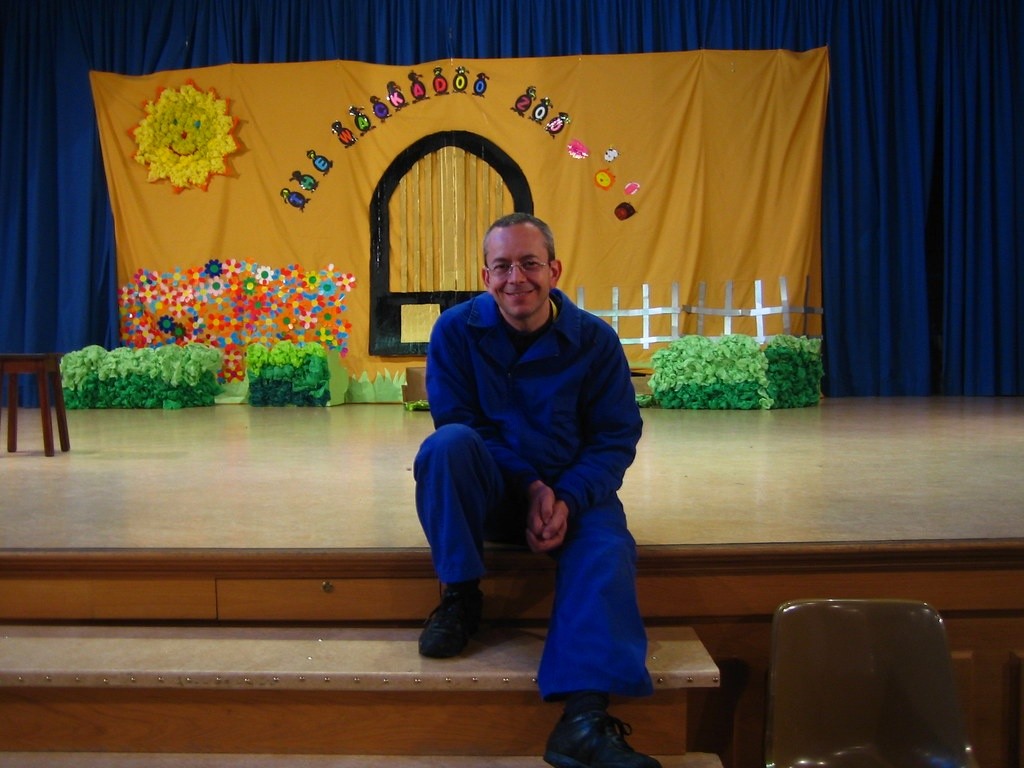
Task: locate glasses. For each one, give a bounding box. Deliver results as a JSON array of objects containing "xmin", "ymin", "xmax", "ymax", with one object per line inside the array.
[{"xmin": 486, "ymin": 259, "xmax": 552, "ymax": 278}]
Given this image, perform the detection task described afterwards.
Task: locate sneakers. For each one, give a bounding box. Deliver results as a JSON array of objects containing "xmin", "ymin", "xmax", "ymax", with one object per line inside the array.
[
  {"xmin": 418, "ymin": 580, "xmax": 484, "ymax": 658},
  {"xmin": 543, "ymin": 709, "xmax": 662, "ymax": 768}
]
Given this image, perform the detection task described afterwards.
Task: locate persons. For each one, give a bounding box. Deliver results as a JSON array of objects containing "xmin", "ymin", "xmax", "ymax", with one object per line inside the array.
[{"xmin": 414, "ymin": 213, "xmax": 660, "ymax": 768}]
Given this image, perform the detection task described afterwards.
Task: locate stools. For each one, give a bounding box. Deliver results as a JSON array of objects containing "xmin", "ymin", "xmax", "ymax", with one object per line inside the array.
[{"xmin": 0, "ymin": 353, "xmax": 70, "ymax": 458}]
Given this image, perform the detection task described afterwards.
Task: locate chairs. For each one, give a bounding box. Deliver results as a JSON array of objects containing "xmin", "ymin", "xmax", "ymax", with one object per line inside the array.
[{"xmin": 761, "ymin": 597, "xmax": 982, "ymax": 768}]
